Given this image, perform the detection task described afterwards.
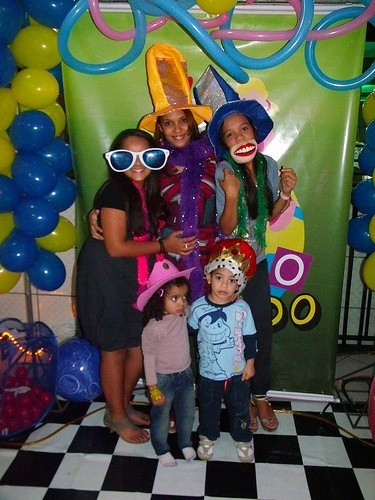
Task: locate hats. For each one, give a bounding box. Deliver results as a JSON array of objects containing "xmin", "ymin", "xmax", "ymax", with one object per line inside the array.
[
  {"xmin": 193, "ymin": 65, "xmax": 274, "ymax": 158},
  {"xmin": 133, "ymin": 254, "xmax": 195, "ymax": 313},
  {"xmin": 203, "ymin": 237, "xmax": 259, "ymax": 296},
  {"xmin": 136, "ymin": 43, "xmax": 212, "ymax": 135}
]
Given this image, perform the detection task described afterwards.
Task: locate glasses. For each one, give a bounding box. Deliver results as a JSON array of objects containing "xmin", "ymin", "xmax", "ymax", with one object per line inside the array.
[{"xmin": 104, "ymin": 147, "xmax": 171, "ymax": 172}]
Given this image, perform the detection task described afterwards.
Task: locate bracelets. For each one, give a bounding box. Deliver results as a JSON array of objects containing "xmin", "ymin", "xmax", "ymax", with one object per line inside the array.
[
  {"xmin": 157, "ymin": 239, "xmax": 165, "ymax": 253},
  {"xmin": 278, "ymin": 192, "xmax": 291, "ymax": 201}
]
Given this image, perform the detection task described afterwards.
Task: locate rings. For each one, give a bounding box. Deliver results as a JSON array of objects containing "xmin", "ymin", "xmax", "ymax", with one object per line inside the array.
[{"xmin": 185, "ymin": 243, "xmax": 188, "ymax": 249}]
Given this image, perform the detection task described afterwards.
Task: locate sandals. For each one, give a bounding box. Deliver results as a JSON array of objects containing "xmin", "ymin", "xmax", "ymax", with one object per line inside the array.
[
  {"xmin": 254, "ymin": 394, "xmax": 280, "ymax": 431},
  {"xmin": 124, "ymin": 405, "xmax": 150, "ymax": 425},
  {"xmin": 104, "ymin": 408, "xmax": 151, "ymax": 444},
  {"xmin": 246, "ymin": 394, "xmax": 259, "ymax": 432}
]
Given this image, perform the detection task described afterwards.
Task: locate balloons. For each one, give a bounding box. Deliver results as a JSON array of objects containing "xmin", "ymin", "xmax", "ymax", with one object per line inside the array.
[
  {"xmin": 0, "ymin": 0, "xmax": 375, "ymax": 296},
  {"xmin": 55, "ymin": 337, "xmax": 101, "ymax": 401},
  {"xmin": 367, "ymin": 377, "xmax": 375, "ymax": 441}
]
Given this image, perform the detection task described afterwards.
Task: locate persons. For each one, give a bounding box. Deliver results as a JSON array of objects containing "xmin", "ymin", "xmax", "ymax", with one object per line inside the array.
[
  {"xmin": 192, "ymin": 63, "xmax": 297, "ymax": 431},
  {"xmin": 88, "ymin": 43, "xmax": 218, "ymax": 305},
  {"xmin": 187, "ymin": 238, "xmax": 258, "ymax": 464},
  {"xmin": 136, "ymin": 259, "xmax": 196, "ymax": 468},
  {"xmin": 75, "ymin": 130, "xmax": 200, "ymax": 444}
]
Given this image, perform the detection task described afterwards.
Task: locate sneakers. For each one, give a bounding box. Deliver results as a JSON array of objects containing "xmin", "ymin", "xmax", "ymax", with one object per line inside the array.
[
  {"xmin": 196, "ymin": 435, "xmax": 215, "ymax": 460},
  {"xmin": 233, "ymin": 440, "xmax": 254, "ymax": 463}
]
